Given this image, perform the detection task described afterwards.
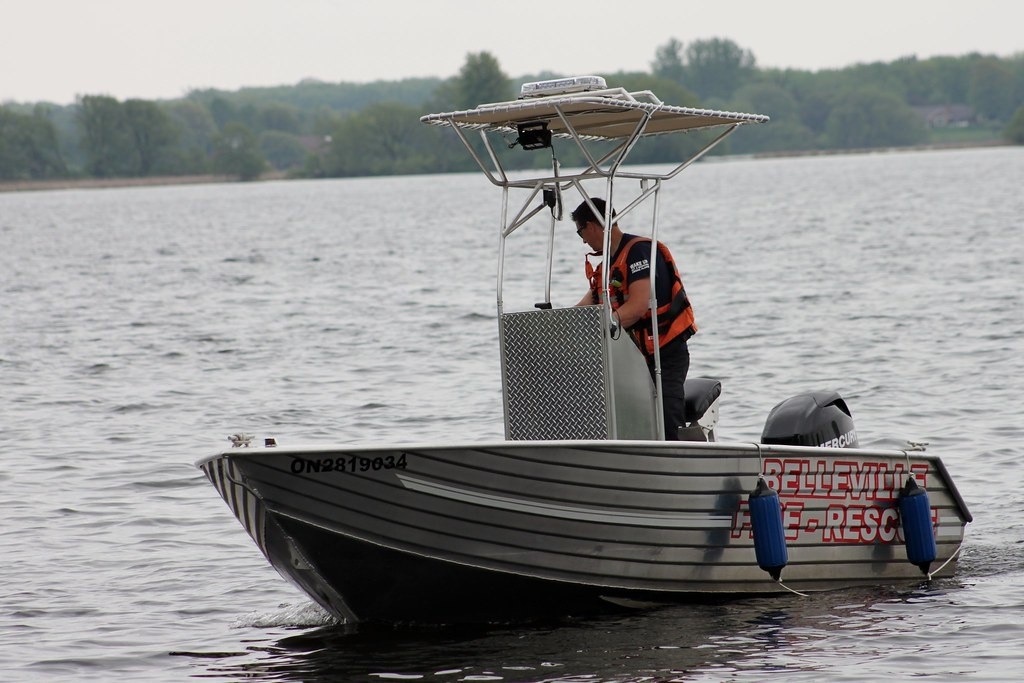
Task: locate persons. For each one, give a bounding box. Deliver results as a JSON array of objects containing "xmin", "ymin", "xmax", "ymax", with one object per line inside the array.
[{"xmin": 571, "ymin": 198, "xmax": 698, "ymax": 441}]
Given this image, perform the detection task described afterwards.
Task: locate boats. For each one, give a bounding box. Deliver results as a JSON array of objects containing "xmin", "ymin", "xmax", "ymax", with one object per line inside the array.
[{"xmin": 195, "ymin": 75, "xmax": 974, "ymax": 625}]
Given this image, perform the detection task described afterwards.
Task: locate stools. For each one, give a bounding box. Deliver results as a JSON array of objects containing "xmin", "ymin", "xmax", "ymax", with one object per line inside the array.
[{"xmin": 681, "ymin": 376, "xmax": 722, "ymax": 442}]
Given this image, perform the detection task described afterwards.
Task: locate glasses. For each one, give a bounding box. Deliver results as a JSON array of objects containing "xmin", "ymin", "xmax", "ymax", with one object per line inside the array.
[{"xmin": 577, "ymin": 221, "xmax": 588, "ymax": 238}]
